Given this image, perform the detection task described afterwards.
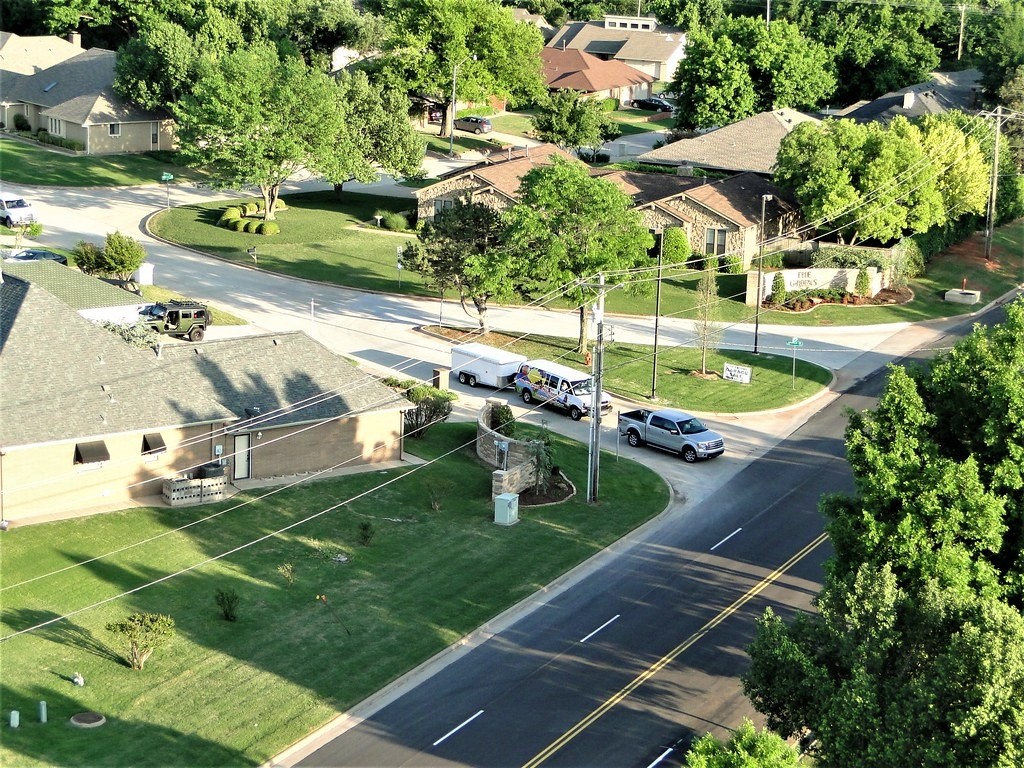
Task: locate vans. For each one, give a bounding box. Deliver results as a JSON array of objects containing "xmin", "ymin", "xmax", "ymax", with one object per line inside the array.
[
  {"xmin": 0, "ymin": 192, "xmax": 33, "ymax": 229},
  {"xmin": 514, "ymin": 359, "xmax": 613, "ymax": 420}
]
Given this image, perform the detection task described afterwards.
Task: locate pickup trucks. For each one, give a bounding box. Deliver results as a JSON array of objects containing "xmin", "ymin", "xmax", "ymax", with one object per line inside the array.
[{"xmin": 619, "ymin": 409, "xmax": 725, "ymax": 462}]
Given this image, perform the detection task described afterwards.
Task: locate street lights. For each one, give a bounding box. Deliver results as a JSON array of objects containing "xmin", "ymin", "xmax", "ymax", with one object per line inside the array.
[
  {"xmin": 751, "ymin": 195, "xmax": 773, "ymax": 355},
  {"xmin": 648, "ymin": 225, "xmax": 663, "ymax": 395},
  {"xmin": 449, "ymin": 54, "xmax": 477, "ymax": 157}
]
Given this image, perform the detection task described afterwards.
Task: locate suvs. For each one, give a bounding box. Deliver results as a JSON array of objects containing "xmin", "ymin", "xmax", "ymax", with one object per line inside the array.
[{"xmin": 139, "ymin": 300, "xmax": 213, "ymax": 342}]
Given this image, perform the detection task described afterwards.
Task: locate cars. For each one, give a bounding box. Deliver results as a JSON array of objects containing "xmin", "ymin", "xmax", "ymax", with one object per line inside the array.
[
  {"xmin": 409, "ymin": 102, "xmax": 440, "ymax": 122},
  {"xmin": 4, "ymin": 249, "xmax": 67, "ymax": 266},
  {"xmin": 454, "ymin": 117, "xmax": 491, "ymax": 134},
  {"xmin": 657, "ymin": 90, "xmax": 694, "ymax": 99},
  {"xmin": 630, "ymin": 98, "xmax": 673, "ymax": 113}
]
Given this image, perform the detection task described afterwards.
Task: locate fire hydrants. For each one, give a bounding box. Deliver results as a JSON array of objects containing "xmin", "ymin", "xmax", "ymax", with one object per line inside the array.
[{"xmin": 585, "ymin": 352, "xmax": 592, "ymax": 365}]
